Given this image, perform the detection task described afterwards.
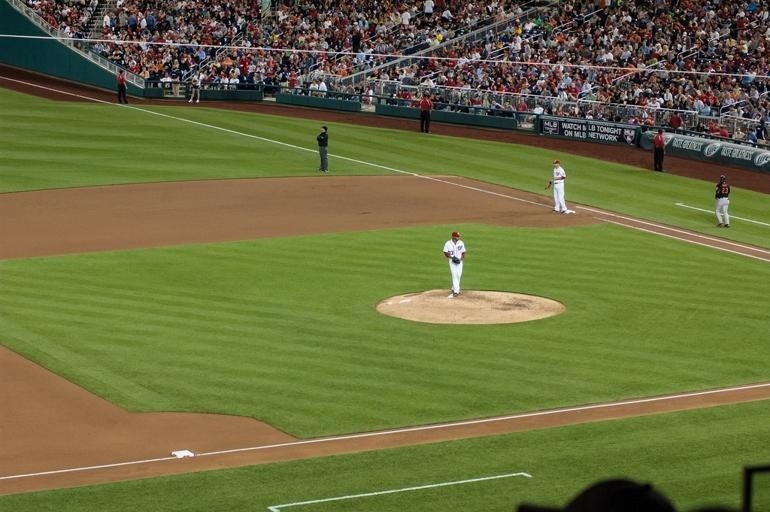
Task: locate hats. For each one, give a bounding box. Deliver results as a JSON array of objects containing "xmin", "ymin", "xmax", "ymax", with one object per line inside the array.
[
  {"xmin": 320, "ymin": 126, "xmax": 328, "ymax": 131},
  {"xmin": 552, "ymin": 159, "xmax": 560, "ymax": 164},
  {"xmin": 452, "ymin": 232, "xmax": 460, "ymax": 237}
]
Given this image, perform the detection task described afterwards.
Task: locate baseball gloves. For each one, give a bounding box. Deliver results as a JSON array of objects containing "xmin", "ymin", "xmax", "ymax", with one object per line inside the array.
[{"xmin": 452, "ymin": 257, "xmax": 460, "ymax": 265}]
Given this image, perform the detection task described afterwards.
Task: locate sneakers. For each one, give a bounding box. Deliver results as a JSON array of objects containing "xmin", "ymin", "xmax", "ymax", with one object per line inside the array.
[
  {"xmin": 718, "ymin": 223, "xmax": 730, "ymax": 228},
  {"xmin": 447, "ymin": 291, "xmax": 463, "ymax": 298}
]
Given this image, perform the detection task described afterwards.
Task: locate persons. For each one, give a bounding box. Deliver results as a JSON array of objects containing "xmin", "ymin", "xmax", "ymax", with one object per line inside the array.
[
  {"xmin": 652, "ymin": 129, "xmax": 666, "ymax": 173},
  {"xmin": 549, "ymin": 160, "xmax": 567, "ymax": 215},
  {"xmin": 443, "ymin": 231, "xmax": 467, "ymax": 298},
  {"xmin": 19, "ymin": 0, "xmax": 770, "ymax": 148},
  {"xmin": 714, "ymin": 175, "xmax": 732, "ymax": 228},
  {"xmin": 317, "ymin": 126, "xmax": 329, "ymax": 172},
  {"xmin": 517, "ymin": 477, "xmax": 676, "ymax": 511}
]
